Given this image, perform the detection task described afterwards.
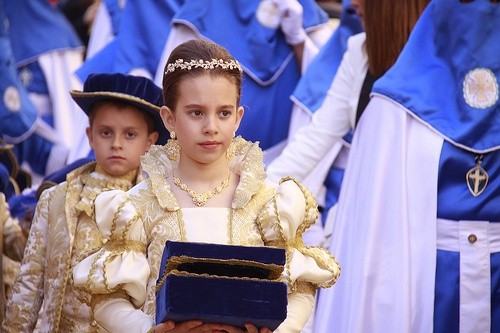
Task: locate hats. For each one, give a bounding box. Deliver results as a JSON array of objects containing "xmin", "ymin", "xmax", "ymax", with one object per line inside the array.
[{"xmin": 69, "ymin": 72, "xmax": 171, "ymax": 145}]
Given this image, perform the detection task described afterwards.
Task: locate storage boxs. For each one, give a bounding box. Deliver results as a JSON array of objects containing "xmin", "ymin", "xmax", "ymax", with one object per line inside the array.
[{"xmin": 156, "ymin": 240, "xmax": 288, "ymax": 332}]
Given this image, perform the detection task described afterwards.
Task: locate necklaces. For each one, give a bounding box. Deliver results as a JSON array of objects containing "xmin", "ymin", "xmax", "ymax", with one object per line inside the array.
[{"xmin": 172, "ymin": 165, "xmax": 234, "ymax": 207}]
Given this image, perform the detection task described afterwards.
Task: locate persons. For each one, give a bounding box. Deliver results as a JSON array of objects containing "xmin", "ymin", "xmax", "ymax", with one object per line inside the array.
[{"xmin": 0, "ymin": 0, "xmax": 500, "ymax": 333}]
